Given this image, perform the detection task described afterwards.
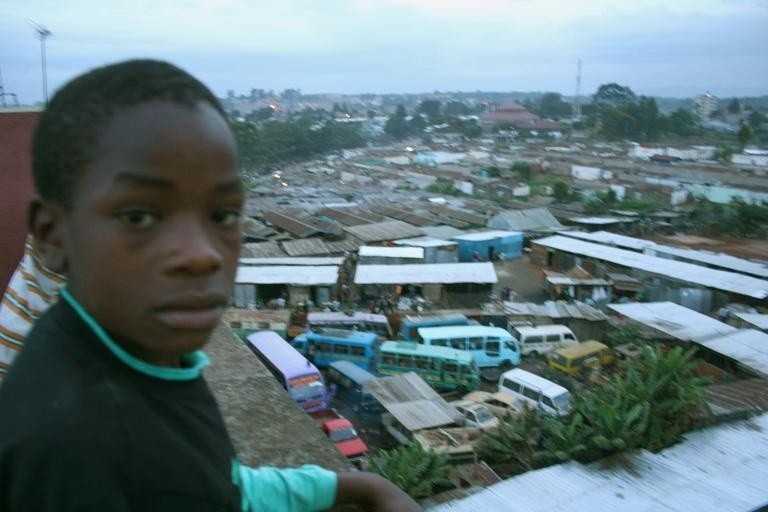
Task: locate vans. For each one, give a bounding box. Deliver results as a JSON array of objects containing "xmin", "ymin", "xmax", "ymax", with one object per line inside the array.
[
  {"xmin": 548, "ymin": 339, "xmax": 616, "ymax": 381},
  {"xmin": 326, "ymin": 361, "xmax": 376, "ymax": 414},
  {"xmin": 508, "ymin": 323, "xmax": 579, "ymax": 359},
  {"xmin": 499, "ymin": 367, "xmax": 575, "ymax": 419}
]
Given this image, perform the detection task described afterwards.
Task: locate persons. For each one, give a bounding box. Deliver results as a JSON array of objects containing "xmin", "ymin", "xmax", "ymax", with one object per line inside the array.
[{"xmin": 1, "ymin": 59, "xmax": 429, "ymax": 511}]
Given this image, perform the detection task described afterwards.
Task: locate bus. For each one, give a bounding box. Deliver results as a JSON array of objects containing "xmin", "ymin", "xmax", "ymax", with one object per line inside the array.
[
  {"xmin": 374, "ymin": 340, "xmax": 482, "ymax": 395},
  {"xmin": 304, "ymin": 308, "xmax": 395, "ymax": 340},
  {"xmin": 396, "ymin": 311, "xmax": 483, "ymax": 342},
  {"xmin": 415, "ymin": 325, "xmax": 522, "ymax": 370},
  {"xmin": 289, "ymin": 326, "xmax": 382, "ymax": 375},
  {"xmin": 246, "ymin": 329, "xmax": 337, "ymax": 414}
]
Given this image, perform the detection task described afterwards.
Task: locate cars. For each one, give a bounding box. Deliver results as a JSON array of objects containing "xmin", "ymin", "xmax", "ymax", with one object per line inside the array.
[
  {"xmin": 461, "ymin": 389, "xmax": 529, "ymax": 419},
  {"xmin": 378, "ymin": 413, "xmax": 414, "ymax": 449},
  {"xmin": 320, "ymin": 416, "xmax": 368, "ymax": 458},
  {"xmin": 449, "ymin": 398, "xmax": 501, "ymax": 436}
]
